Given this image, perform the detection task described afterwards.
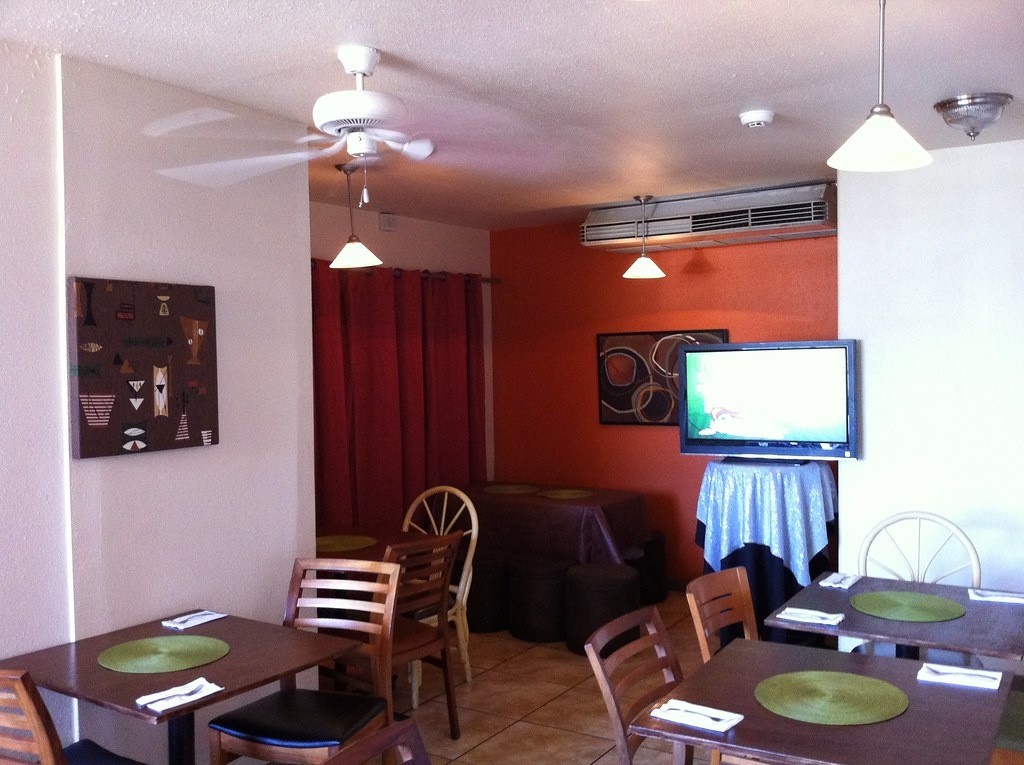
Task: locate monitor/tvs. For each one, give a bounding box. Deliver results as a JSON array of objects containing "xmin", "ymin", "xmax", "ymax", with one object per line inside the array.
[{"xmin": 677, "ymin": 338, "xmax": 859, "ymax": 465}]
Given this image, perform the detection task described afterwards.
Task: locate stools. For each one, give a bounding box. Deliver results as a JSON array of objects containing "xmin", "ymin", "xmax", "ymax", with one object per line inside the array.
[{"xmin": 450, "ymin": 528, "xmax": 668, "ymax": 658}]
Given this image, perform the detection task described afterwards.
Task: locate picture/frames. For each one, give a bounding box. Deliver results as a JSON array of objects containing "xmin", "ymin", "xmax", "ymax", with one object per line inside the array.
[{"xmin": 597, "ymin": 329, "xmax": 728, "ymax": 426}]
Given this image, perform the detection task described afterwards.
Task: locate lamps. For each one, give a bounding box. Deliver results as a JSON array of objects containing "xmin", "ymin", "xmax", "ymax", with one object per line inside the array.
[
  {"xmin": 621, "ymin": 195, "xmax": 666, "ymax": 279},
  {"xmin": 933, "ymin": 94, "xmax": 1013, "ymax": 142},
  {"xmin": 328, "ymin": 164, "xmax": 383, "ymax": 269},
  {"xmin": 826, "ymin": 0, "xmax": 935, "ymax": 172}
]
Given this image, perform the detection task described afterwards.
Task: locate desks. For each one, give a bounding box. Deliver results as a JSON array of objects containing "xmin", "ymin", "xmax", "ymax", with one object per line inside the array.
[
  {"xmin": 628, "ymin": 638, "xmax": 1015, "ymax": 765},
  {"xmin": 694, "ymin": 456, "xmax": 838, "ymax": 648},
  {"xmin": 452, "ymin": 481, "xmax": 644, "ymax": 567},
  {"xmin": 0, "ymin": 608, "xmax": 364, "ymax": 765},
  {"xmin": 316, "ymin": 524, "xmax": 442, "ymax": 723},
  {"xmin": 763, "ymin": 571, "xmax": 1024, "ymax": 663}
]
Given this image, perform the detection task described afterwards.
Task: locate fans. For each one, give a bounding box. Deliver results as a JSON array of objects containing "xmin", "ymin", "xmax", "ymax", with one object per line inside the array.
[{"xmin": 142, "ymin": 42, "xmax": 543, "ymax": 206}]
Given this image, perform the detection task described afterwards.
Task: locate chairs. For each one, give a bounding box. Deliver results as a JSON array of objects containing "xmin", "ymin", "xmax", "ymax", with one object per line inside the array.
[
  {"xmin": 858, "ymin": 511, "xmax": 981, "ymax": 588},
  {"xmin": 584, "ymin": 604, "xmax": 684, "ymax": 765},
  {"xmin": 318, "ymin": 717, "xmax": 432, "ymax": 765},
  {"xmin": 0, "ymin": 669, "xmax": 148, "ymax": 765},
  {"xmin": 208, "ymin": 559, "xmax": 400, "ymax": 765},
  {"xmin": 319, "ymin": 530, "xmax": 464, "ymax": 740},
  {"xmin": 685, "ymin": 566, "xmax": 773, "ymax": 765}
]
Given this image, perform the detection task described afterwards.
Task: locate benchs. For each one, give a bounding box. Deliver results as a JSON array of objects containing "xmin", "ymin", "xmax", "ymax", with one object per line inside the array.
[{"xmin": 402, "ymin": 485, "xmax": 480, "ymax": 709}]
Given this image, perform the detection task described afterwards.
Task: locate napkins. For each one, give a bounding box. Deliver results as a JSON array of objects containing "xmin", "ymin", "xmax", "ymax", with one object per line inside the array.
[
  {"xmin": 650, "ymin": 698, "xmax": 744, "ymax": 732},
  {"xmin": 136, "ymin": 677, "xmax": 225, "ymax": 713},
  {"xmin": 819, "ymin": 572, "xmax": 862, "ymax": 589},
  {"xmin": 161, "ymin": 611, "xmax": 229, "ymax": 630},
  {"xmin": 967, "ymin": 588, "xmax": 1024, "ymax": 604},
  {"xmin": 776, "ymin": 607, "xmax": 846, "ymax": 625},
  {"xmin": 917, "ymin": 662, "xmax": 1003, "ymax": 689}
]
